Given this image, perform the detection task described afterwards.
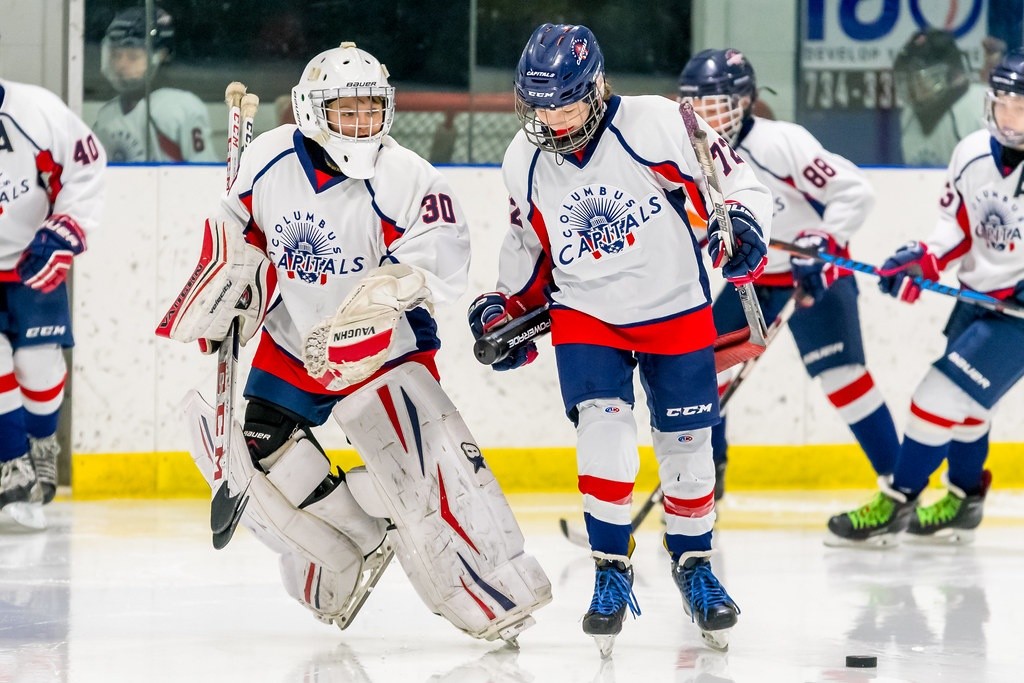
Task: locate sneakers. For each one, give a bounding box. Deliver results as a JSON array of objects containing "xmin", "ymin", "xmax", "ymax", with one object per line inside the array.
[
  {"xmin": 30, "ymin": 432, "xmax": 61, "ymax": 504},
  {"xmin": 0, "ymin": 453, "xmax": 48, "ymax": 530},
  {"xmin": 663, "ymin": 532, "xmax": 741, "ymax": 651},
  {"xmin": 583, "ymin": 533, "xmax": 640, "ymax": 659},
  {"xmin": 823, "ymin": 476, "xmax": 928, "ymax": 550},
  {"xmin": 906, "ymin": 470, "xmax": 992, "ymax": 542}
]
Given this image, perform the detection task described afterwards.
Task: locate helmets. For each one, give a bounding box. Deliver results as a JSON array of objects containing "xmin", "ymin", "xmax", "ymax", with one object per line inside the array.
[
  {"xmin": 989, "ymin": 46, "xmax": 1024, "ymax": 95},
  {"xmin": 512, "ymin": 22, "xmax": 606, "ymax": 155},
  {"xmin": 678, "ymin": 49, "xmax": 757, "ymax": 151},
  {"xmin": 291, "ymin": 42, "xmax": 395, "ymax": 180},
  {"xmin": 894, "ymin": 31, "xmax": 968, "ymax": 134},
  {"xmin": 94, "ymin": 6, "xmax": 175, "ymax": 96}
]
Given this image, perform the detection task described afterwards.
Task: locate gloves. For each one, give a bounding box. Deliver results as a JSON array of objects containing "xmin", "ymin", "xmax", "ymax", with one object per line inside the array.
[
  {"xmin": 707, "ymin": 205, "xmax": 767, "ymax": 288},
  {"xmin": 790, "ymin": 231, "xmax": 839, "ymax": 300},
  {"xmin": 17, "ymin": 215, "xmax": 88, "ymax": 294},
  {"xmin": 878, "ymin": 243, "xmax": 938, "ymax": 303},
  {"xmin": 466, "ymin": 292, "xmax": 538, "ymax": 371}
]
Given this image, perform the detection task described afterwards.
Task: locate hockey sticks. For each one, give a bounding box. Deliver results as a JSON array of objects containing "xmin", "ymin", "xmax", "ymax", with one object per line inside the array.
[
  {"xmin": 472, "ymin": 304, "xmax": 558, "ymax": 368},
  {"xmin": 762, "ymin": 234, "xmax": 1024, "ymax": 329},
  {"xmin": 678, "ymin": 97, "xmax": 771, "ymax": 378},
  {"xmin": 200, "ymin": 78, "xmax": 264, "ymax": 552},
  {"xmin": 552, "ymin": 244, "xmax": 821, "ymax": 556}
]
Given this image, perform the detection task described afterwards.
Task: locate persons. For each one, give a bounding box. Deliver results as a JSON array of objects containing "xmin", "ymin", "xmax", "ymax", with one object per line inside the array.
[
  {"xmin": 89, "ymin": 7, "xmax": 216, "ymax": 164},
  {"xmin": 0, "ymin": 78, "xmax": 106, "ymax": 528},
  {"xmin": 827, "ymin": 47, "xmax": 1024, "ymax": 547},
  {"xmin": 679, "ymin": 49, "xmax": 900, "ymax": 485},
  {"xmin": 894, "ymin": 28, "xmax": 990, "ymax": 164},
  {"xmin": 152, "ymin": 42, "xmax": 547, "ymax": 650},
  {"xmin": 466, "ymin": 26, "xmax": 768, "ymax": 652}
]
